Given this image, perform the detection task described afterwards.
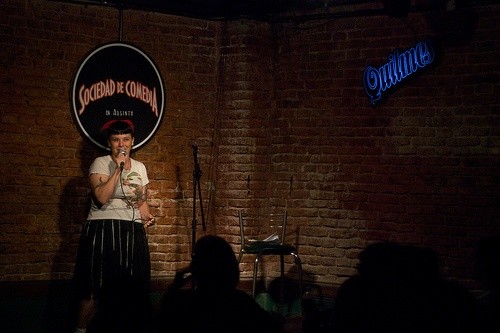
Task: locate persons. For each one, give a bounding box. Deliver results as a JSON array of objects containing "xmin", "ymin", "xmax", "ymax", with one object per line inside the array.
[
  {"xmin": 70, "ymin": 119, "xmax": 153, "ymax": 331},
  {"xmin": 327, "ymin": 242, "xmax": 454, "ymax": 332},
  {"xmin": 156, "ymin": 232, "xmax": 285, "ymax": 332}
]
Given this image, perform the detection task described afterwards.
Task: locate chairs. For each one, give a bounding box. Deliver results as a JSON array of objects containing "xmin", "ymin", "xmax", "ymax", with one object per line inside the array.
[{"xmin": 236, "ymin": 206, "xmax": 303, "ymax": 302}]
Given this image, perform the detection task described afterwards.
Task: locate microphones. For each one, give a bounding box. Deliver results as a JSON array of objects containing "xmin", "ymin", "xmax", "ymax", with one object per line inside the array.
[{"xmin": 119, "ymin": 148, "xmax": 126, "ymax": 171}]
